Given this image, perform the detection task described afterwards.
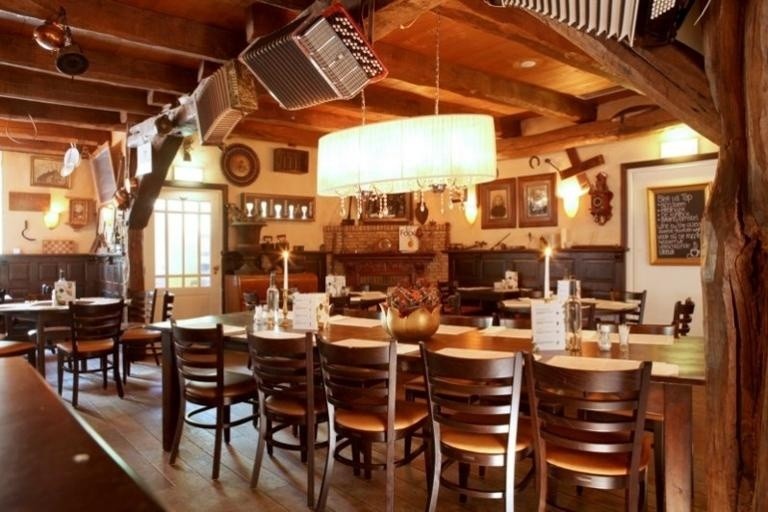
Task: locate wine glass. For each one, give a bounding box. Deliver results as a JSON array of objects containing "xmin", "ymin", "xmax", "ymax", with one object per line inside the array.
[{"xmin": 247, "ymin": 201, "xmax": 308, "ymax": 219}]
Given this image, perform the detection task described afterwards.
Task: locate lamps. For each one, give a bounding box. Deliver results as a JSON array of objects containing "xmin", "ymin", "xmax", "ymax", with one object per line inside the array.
[{"xmin": 316, "ymin": 0, "xmax": 497, "ymax": 221}]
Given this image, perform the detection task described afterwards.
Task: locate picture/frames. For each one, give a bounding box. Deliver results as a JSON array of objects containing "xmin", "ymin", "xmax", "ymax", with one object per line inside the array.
[
  {"xmin": 30, "ymin": 155, "xmax": 73, "ymax": 190},
  {"xmin": 647, "ymin": 182, "xmax": 711, "ymax": 266},
  {"xmin": 517, "ymin": 171, "xmax": 558, "ymax": 228},
  {"xmin": 481, "ymin": 177, "xmax": 516, "ymax": 230},
  {"xmin": 361, "ymin": 189, "xmax": 413, "ymax": 225}
]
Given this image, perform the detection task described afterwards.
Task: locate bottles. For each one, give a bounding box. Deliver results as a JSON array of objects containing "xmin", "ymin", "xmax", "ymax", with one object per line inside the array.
[
  {"xmin": 266, "ymin": 273, "xmax": 279, "ymax": 321},
  {"xmin": 565, "ymin": 280, "xmax": 582, "ymax": 352},
  {"xmin": 54, "ymin": 268, "xmax": 65, "ymax": 304}
]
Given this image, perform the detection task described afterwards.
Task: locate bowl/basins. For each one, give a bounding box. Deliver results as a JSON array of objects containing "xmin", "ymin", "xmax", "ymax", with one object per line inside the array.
[{"xmin": 475, "ymin": 317, "xmax": 494, "ymax": 329}]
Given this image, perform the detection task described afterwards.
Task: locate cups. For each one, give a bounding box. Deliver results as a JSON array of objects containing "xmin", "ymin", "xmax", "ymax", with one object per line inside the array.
[
  {"xmin": 597, "ymin": 323, "xmax": 611, "ymax": 350},
  {"xmin": 618, "ymin": 325, "xmax": 632, "ymax": 351}
]
